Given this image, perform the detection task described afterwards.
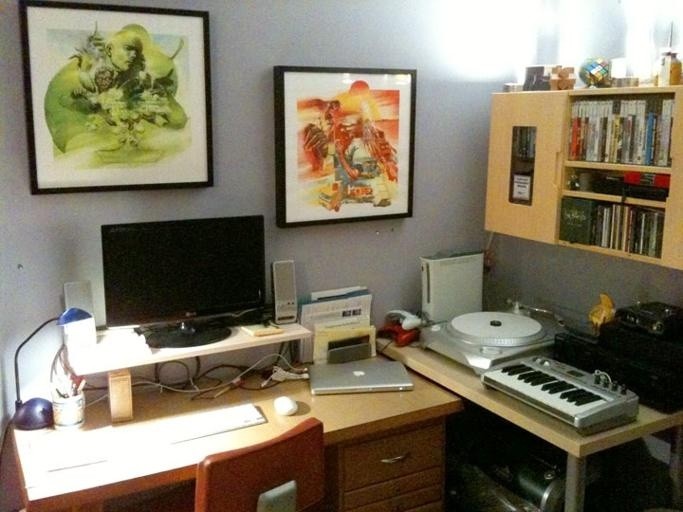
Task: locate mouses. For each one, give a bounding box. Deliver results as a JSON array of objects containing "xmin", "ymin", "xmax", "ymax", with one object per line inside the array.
[{"xmin": 274, "ymin": 395, "xmax": 298, "ymax": 416}]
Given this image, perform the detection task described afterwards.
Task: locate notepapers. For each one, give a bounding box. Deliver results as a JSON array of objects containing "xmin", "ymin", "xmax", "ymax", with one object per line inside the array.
[{"xmin": 240, "ymin": 324, "xmax": 283, "ymax": 337}]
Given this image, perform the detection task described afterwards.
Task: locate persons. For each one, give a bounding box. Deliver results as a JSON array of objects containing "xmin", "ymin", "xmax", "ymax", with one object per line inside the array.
[{"xmin": 44, "ymin": 32, "xmax": 187, "ymax": 152}]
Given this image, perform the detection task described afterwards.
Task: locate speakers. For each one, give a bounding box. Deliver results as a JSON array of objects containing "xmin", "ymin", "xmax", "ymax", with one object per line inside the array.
[
  {"xmin": 272, "ymin": 258, "xmax": 297, "ymax": 324},
  {"xmin": 63, "ymin": 281, "xmax": 98, "ymax": 348}
]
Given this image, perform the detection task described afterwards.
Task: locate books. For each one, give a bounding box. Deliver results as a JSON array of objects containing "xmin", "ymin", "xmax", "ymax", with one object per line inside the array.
[{"xmin": 561, "ymin": 98, "xmax": 673, "ymax": 257}]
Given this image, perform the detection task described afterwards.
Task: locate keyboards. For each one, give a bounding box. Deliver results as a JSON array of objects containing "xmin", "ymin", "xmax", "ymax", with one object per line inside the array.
[{"xmin": 161, "ymin": 402, "xmax": 267, "ymax": 443}]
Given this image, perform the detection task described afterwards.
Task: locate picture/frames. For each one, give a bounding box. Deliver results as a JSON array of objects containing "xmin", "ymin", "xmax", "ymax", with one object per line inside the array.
[
  {"xmin": 272, "ymin": 64, "xmax": 417, "ymax": 229},
  {"xmin": 12, "ymin": 0, "xmax": 215, "ymax": 195}
]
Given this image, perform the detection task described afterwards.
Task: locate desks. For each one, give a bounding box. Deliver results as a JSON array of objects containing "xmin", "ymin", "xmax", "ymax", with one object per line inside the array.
[
  {"xmin": 11, "ymin": 355, "xmax": 462, "ymax": 511},
  {"xmin": 370, "ymin": 331, "xmax": 682, "ymax": 512}
]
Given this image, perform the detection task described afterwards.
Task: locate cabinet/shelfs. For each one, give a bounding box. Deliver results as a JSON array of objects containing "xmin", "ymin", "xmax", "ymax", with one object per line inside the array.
[
  {"xmin": 483, "ymin": 90, "xmax": 566, "ymax": 247},
  {"xmin": 557, "ymin": 89, "xmax": 674, "ymax": 271}
]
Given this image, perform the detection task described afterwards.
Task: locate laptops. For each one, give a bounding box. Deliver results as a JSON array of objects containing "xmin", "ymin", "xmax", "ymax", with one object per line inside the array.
[{"xmin": 309, "ymin": 360, "xmax": 413, "ymax": 394}]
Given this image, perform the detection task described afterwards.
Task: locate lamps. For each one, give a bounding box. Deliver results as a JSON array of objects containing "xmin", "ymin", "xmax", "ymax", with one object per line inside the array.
[{"xmin": 10, "ymin": 304, "xmax": 91, "ymax": 431}]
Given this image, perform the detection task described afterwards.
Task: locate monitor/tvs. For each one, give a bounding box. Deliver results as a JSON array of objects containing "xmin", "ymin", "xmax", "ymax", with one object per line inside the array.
[{"xmin": 100, "ymin": 215, "xmax": 265, "ymax": 348}]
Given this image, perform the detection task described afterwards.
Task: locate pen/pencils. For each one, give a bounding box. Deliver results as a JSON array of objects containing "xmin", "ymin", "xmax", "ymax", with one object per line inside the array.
[{"xmin": 55, "ymin": 373, "xmax": 86, "ymax": 398}]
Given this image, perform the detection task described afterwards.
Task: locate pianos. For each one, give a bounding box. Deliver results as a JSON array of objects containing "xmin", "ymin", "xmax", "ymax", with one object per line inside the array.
[{"xmin": 482, "ymin": 355, "xmax": 638, "ymax": 436}]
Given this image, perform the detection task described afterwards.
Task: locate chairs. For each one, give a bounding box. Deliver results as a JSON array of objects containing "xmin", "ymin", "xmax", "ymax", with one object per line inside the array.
[{"xmin": 192, "ymin": 416, "xmax": 326, "ymax": 510}]
